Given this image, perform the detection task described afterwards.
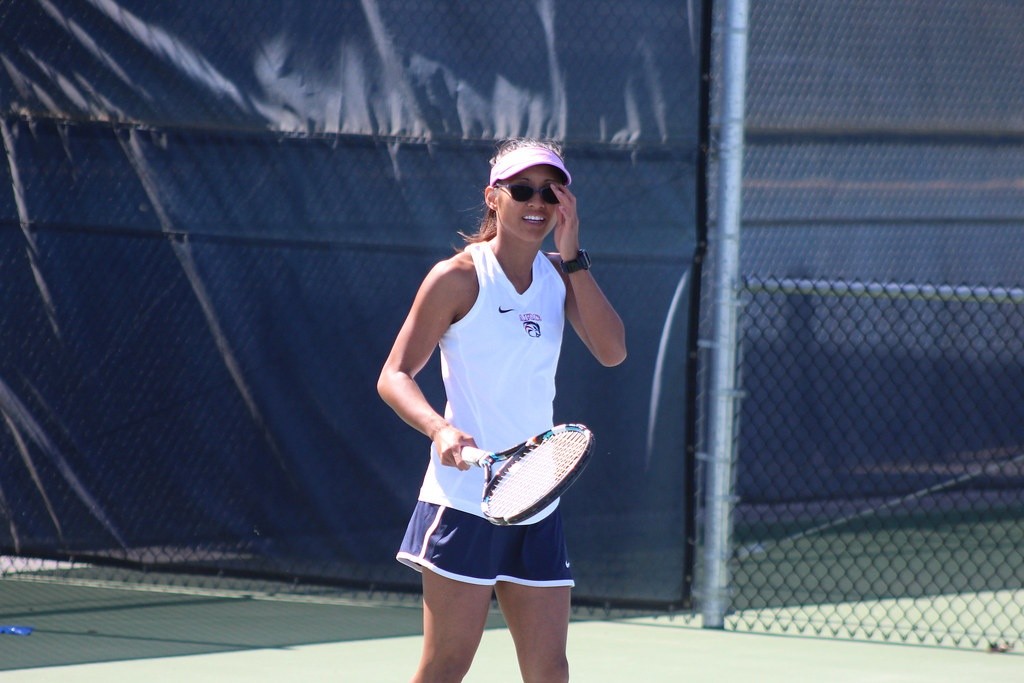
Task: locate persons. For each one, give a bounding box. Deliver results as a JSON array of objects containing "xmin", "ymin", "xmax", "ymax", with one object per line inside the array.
[{"xmin": 376, "ymin": 140, "xmax": 627, "ymax": 682}]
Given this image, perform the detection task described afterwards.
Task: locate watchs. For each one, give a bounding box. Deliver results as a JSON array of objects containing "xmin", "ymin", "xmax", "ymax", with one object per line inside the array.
[{"xmin": 560, "ymin": 247, "xmax": 592, "ymax": 274}]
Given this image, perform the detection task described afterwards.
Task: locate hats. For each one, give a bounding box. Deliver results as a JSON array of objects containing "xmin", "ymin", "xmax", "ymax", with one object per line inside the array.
[{"xmin": 490, "ymin": 147, "xmax": 572, "ymax": 186}]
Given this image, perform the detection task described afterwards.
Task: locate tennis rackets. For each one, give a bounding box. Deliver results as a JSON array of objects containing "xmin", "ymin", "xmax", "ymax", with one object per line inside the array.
[{"xmin": 460, "ymin": 422, "xmax": 596, "ymax": 526}]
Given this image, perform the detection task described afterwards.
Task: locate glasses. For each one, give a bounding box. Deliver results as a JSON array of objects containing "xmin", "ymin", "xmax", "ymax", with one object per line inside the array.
[{"xmin": 495, "ymin": 181, "xmax": 560, "ymax": 205}]
